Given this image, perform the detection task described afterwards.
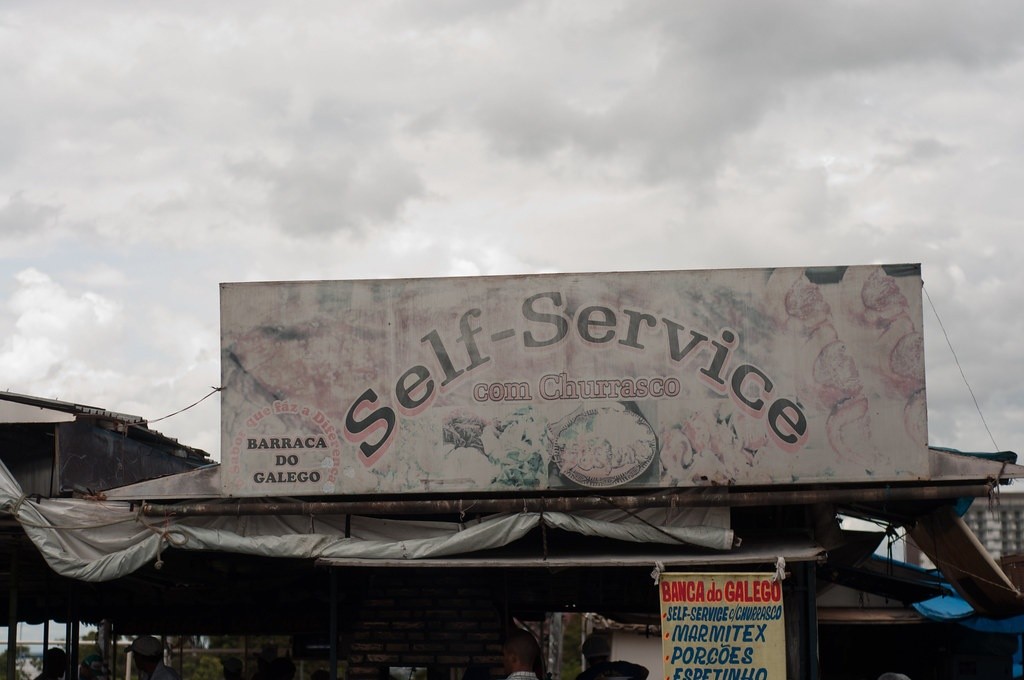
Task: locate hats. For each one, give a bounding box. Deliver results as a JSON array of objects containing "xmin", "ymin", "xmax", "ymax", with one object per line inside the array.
[
  {"xmin": 221, "ymin": 656, "xmax": 242, "ymax": 675},
  {"xmin": 83, "ymin": 654, "xmax": 103, "ymax": 675},
  {"xmin": 124, "ymin": 635, "xmax": 161, "ymax": 656},
  {"xmin": 252, "ymin": 642, "xmax": 277, "ymax": 662},
  {"xmin": 582, "ymin": 634, "xmax": 611, "ymax": 657}
]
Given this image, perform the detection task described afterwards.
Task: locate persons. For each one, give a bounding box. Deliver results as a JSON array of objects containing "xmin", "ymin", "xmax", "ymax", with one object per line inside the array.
[
  {"xmin": 77, "ymin": 654, "xmax": 112, "ymax": 680},
  {"xmin": 575, "ymin": 636, "xmax": 649, "ymax": 680},
  {"xmin": 33, "ymin": 648, "xmax": 67, "ymax": 680},
  {"xmin": 124, "ymin": 637, "xmax": 183, "ymax": 680},
  {"xmin": 222, "ymin": 648, "xmax": 331, "ymax": 680},
  {"xmin": 502, "ymin": 632, "xmax": 540, "ymax": 680}
]
